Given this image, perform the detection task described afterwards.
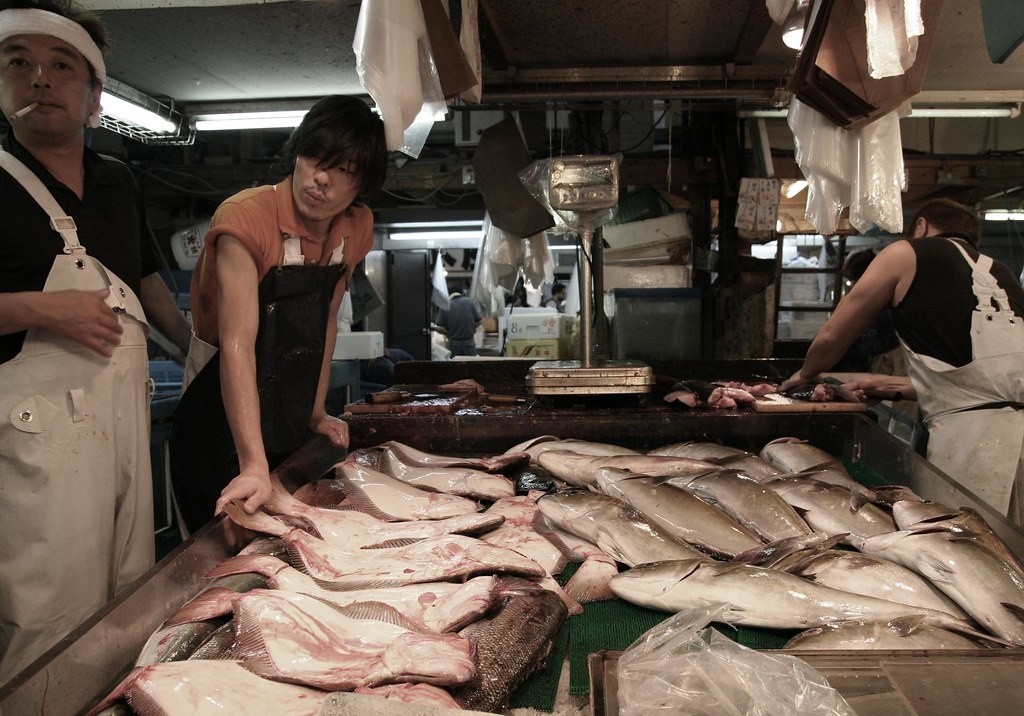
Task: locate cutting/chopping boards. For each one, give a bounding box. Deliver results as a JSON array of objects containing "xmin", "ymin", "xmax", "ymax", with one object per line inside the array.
[
  {"xmin": 751, "ymin": 392, "xmax": 868, "ymax": 412},
  {"xmin": 344, "ymin": 384, "xmax": 478, "ymax": 418}
]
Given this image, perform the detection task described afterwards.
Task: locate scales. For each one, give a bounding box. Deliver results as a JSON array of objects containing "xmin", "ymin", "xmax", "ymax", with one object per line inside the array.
[{"xmin": 524, "ymin": 155, "xmax": 656, "ymax": 399}]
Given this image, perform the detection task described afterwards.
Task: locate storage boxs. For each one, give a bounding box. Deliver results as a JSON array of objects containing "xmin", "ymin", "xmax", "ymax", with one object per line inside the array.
[
  {"xmin": 506, "ymin": 314, "xmax": 574, "ymax": 361},
  {"xmin": 330, "ymin": 330, "xmax": 384, "ymax": 359},
  {"xmin": 604, "ymin": 213, "xmax": 693, "ymax": 287}
]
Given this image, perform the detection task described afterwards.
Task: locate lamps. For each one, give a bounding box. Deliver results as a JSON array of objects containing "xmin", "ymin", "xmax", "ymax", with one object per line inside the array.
[{"xmin": 781, "ymin": 0, "xmax": 811, "ymax": 50}]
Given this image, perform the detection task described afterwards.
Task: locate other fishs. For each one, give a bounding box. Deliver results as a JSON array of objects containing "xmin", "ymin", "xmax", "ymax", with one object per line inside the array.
[
  {"xmin": 84, "ymin": 435, "xmax": 1023, "ymax": 716},
  {"xmin": 663, "ymin": 378, "xmax": 867, "ymax": 411}
]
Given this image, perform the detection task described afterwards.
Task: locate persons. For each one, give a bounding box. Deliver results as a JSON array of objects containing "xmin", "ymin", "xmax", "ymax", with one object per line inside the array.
[
  {"xmin": 0, "ymin": 0, "xmax": 191, "ymax": 689},
  {"xmin": 167, "ymin": 94, "xmax": 388, "ymax": 541},
  {"xmin": 436, "ymin": 287, "xmax": 482, "ymax": 357},
  {"xmin": 543, "ymin": 285, "xmax": 567, "ymax": 312},
  {"xmin": 777, "ymin": 198, "xmax": 1024, "ymax": 531},
  {"xmin": 842, "ymin": 244, "xmax": 920, "ymax": 450}
]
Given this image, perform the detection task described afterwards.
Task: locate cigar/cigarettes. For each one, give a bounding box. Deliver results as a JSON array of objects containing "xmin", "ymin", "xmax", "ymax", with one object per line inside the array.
[{"xmin": 9, "ymin": 103, "xmax": 39, "ymax": 121}]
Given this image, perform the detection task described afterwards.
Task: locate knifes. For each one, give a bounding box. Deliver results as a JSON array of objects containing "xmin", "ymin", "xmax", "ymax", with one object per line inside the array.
[{"xmin": 365, "ymin": 391, "xmax": 440, "ymax": 404}]
